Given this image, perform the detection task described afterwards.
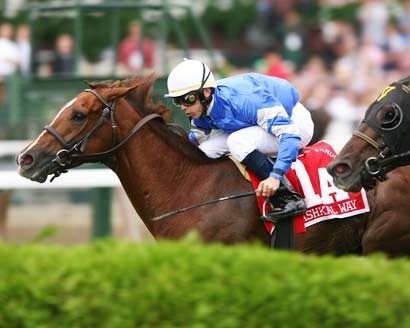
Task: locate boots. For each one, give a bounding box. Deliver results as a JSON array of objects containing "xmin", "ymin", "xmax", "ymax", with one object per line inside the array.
[{"xmin": 240, "ymin": 150, "xmax": 307, "ymax": 221}]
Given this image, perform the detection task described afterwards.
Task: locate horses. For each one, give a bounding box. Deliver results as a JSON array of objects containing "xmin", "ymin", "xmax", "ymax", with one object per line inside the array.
[
  {"xmin": 15, "ymin": 73, "xmax": 374, "ymax": 256},
  {"xmin": 326, "ymin": 75, "xmax": 410, "ymax": 193}
]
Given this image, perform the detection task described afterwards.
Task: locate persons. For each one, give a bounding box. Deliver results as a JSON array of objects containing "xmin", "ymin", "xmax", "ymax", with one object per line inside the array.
[
  {"xmin": 227, "ymin": 0, "xmax": 409, "ymax": 157},
  {"xmin": 0, "ymin": 16, "xmax": 161, "ymax": 78},
  {"xmin": 163, "ymin": 58, "xmax": 315, "ymax": 222}
]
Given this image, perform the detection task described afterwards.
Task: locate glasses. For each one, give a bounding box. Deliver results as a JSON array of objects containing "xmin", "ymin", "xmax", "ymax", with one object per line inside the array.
[{"xmin": 172, "ymin": 87, "xmax": 202, "ymax": 107}]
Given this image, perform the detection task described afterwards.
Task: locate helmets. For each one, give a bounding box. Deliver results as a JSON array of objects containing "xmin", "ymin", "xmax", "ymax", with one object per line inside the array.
[{"xmin": 164, "ymin": 57, "xmax": 217, "ymax": 98}]
